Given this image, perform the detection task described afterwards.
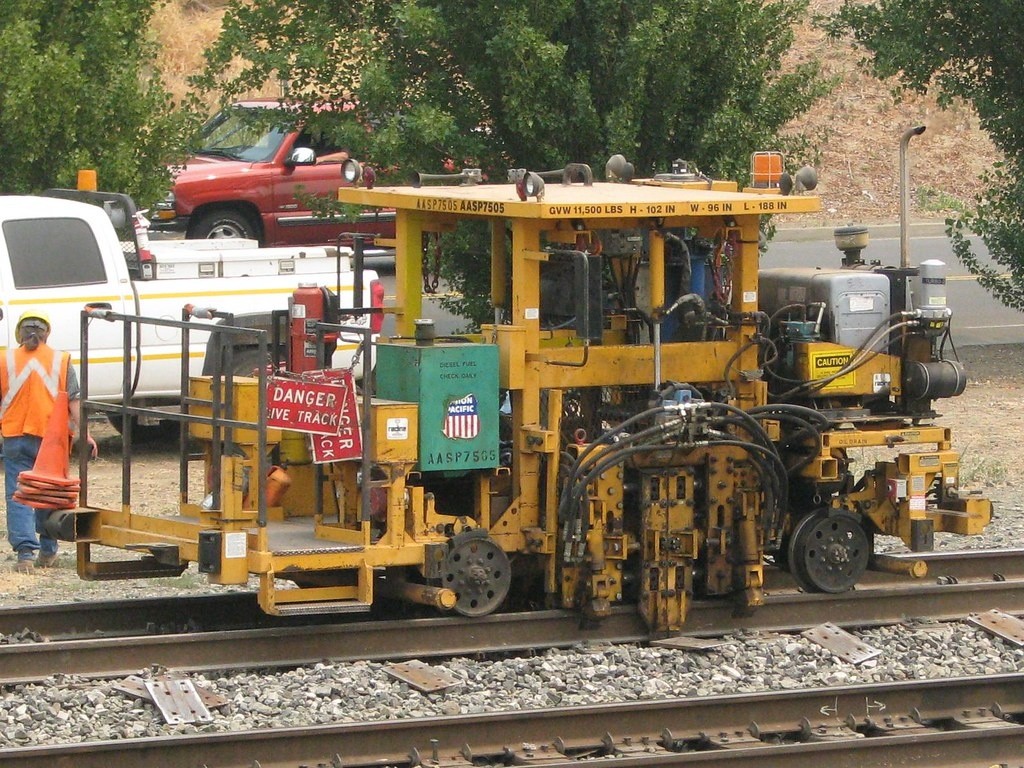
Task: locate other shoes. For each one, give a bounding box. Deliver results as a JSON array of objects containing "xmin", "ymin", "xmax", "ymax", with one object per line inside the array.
[
  {"xmin": 38, "ymin": 551, "xmax": 59, "ymax": 567},
  {"xmin": 13, "ymin": 560, "xmax": 34, "ymax": 575}
]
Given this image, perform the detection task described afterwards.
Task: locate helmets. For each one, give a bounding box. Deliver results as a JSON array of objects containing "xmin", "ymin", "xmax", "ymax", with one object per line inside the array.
[{"xmin": 15, "ymin": 312, "xmax": 51, "ymax": 344}]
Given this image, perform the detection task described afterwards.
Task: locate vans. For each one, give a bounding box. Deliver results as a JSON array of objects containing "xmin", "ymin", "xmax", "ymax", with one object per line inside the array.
[{"xmin": 140, "ymin": 97, "xmax": 508, "ymax": 250}]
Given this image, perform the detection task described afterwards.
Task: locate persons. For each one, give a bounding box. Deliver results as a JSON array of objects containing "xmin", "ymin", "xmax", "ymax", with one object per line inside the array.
[{"xmin": 0, "ymin": 308, "xmax": 98, "ymax": 575}]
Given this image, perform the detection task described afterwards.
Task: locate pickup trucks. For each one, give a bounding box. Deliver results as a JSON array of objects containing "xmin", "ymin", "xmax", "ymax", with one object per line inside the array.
[{"xmin": 0, "ymin": 187, "xmax": 384, "ymax": 445}]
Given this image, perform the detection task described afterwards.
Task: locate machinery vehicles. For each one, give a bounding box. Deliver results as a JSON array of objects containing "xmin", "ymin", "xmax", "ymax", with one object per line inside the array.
[{"xmin": 33, "ymin": 123, "xmax": 996, "ymax": 643}]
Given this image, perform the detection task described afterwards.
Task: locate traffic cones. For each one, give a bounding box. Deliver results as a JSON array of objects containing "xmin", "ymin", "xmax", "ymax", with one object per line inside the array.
[{"xmin": 12, "ymin": 391, "xmax": 82, "ymax": 511}]
